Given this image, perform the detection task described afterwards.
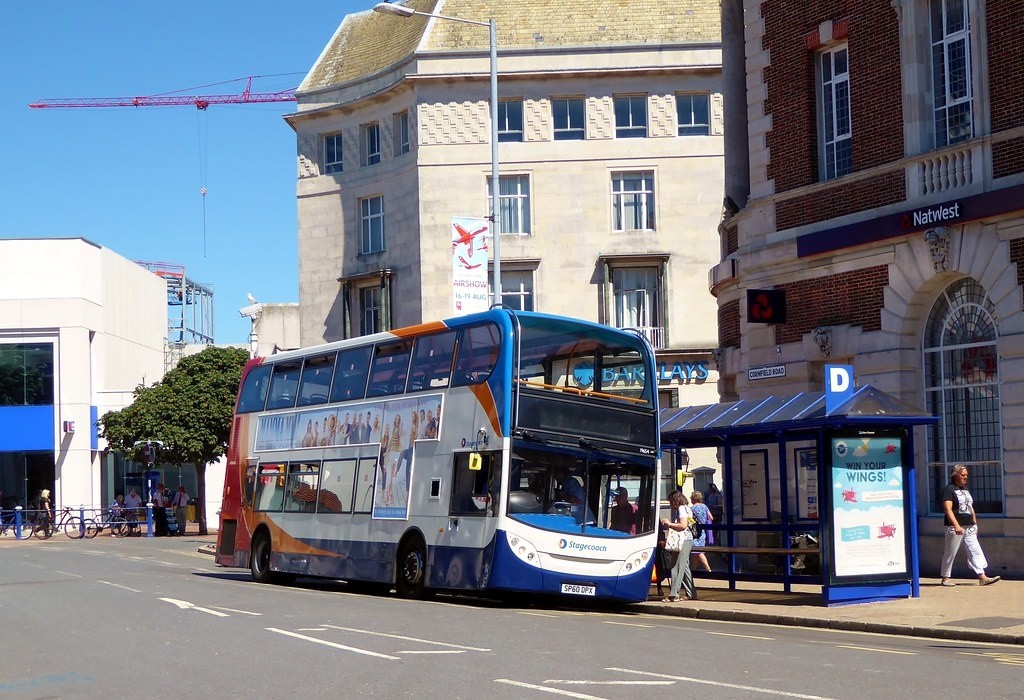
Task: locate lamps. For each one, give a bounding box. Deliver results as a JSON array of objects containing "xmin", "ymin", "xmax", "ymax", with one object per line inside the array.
[{"xmin": 723, "ymin": 197, "xmax": 739, "ymax": 215}]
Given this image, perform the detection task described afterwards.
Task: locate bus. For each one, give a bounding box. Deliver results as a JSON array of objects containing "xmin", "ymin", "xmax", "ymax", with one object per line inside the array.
[{"xmin": 213, "ymin": 303, "xmax": 695, "ymax": 602}]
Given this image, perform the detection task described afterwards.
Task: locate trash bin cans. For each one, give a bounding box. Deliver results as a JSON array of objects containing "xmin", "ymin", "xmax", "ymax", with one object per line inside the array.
[{"xmin": 758, "ymin": 518, "xmax": 781, "ymax": 573}]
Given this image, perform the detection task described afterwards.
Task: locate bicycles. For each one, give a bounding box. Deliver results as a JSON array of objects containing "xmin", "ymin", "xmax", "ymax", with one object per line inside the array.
[
  {"xmin": 33, "ymin": 507, "xmax": 85, "ymax": 540},
  {"xmin": 84, "ymin": 508, "xmax": 131, "ymax": 538},
  {"xmin": 0, "ymin": 507, "xmax": 34, "ymax": 540}
]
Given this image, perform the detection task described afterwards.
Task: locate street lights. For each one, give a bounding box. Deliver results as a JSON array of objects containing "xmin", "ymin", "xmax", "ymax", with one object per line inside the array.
[
  {"xmin": 134, "ymin": 438, "xmax": 164, "ymax": 503},
  {"xmin": 372, "ymin": 1, "xmax": 503, "ymax": 310}
]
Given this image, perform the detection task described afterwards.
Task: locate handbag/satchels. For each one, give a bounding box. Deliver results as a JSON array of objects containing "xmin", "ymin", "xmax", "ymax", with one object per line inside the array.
[
  {"xmin": 664, "ymin": 528, "xmax": 685, "ymax": 552},
  {"xmin": 692, "ymin": 513, "xmax": 702, "ymax": 539},
  {"xmin": 657, "ymin": 550, "xmax": 672, "ymax": 569},
  {"xmin": 705, "ymin": 514, "xmax": 714, "ymax": 544}
]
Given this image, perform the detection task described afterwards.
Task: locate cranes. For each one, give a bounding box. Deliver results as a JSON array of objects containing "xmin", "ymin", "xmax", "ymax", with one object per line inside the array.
[{"xmin": 26, "ymin": 75, "xmax": 301, "ymax": 260}]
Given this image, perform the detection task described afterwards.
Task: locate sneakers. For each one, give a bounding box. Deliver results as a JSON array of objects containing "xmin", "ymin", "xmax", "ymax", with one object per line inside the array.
[
  {"xmin": 680, "ymin": 595, "xmax": 698, "ymax": 600},
  {"xmin": 662, "ymin": 596, "xmax": 679, "ymax": 603}
]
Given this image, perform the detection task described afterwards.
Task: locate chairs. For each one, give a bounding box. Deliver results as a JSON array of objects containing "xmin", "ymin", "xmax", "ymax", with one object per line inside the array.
[{"xmin": 275, "ymin": 370, "xmax": 492, "ymax": 408}]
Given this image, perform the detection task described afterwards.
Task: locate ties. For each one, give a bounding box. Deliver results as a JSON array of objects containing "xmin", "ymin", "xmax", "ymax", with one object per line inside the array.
[{"xmin": 177, "ymin": 493, "xmax": 182, "ymax": 506}]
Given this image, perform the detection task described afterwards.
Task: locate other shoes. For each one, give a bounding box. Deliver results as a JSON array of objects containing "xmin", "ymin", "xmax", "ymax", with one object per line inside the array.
[
  {"xmin": 979, "ymin": 576, "xmax": 1000, "ymax": 586},
  {"xmin": 941, "ymin": 580, "xmax": 955, "ymax": 587}
]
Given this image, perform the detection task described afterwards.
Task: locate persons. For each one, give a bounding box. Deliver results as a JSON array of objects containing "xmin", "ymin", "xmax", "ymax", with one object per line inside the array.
[
  {"xmin": 556, "ymin": 469, "xmax": 596, "ymax": 526},
  {"xmin": 482, "ymin": 467, "xmax": 500, "ymax": 496},
  {"xmin": 941, "ymin": 465, "xmax": 1001, "ymax": 586},
  {"xmin": 609, "ymin": 488, "xmax": 714, "ymax": 603},
  {"xmin": 705, "ymin": 485, "xmax": 722, "ymax": 542},
  {"xmin": 154, "ymin": 484, "xmax": 171, "ymax": 535},
  {"xmin": 171, "ymin": 486, "xmax": 189, "ymax": 535},
  {"xmin": 791, "ymin": 529, "xmax": 820, "ymax": 568},
  {"xmin": 302, "ymin": 405, "xmax": 441, "ymax": 505},
  {"xmin": 35, "ymin": 490, "xmax": 52, "ymax": 537},
  {"xmin": 111, "ymin": 490, "xmax": 142, "ymax": 534}
]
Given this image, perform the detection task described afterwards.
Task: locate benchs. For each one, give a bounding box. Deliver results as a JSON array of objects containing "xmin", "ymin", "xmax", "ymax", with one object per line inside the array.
[{"xmin": 688, "ymin": 546, "xmax": 822, "ymax": 554}]
